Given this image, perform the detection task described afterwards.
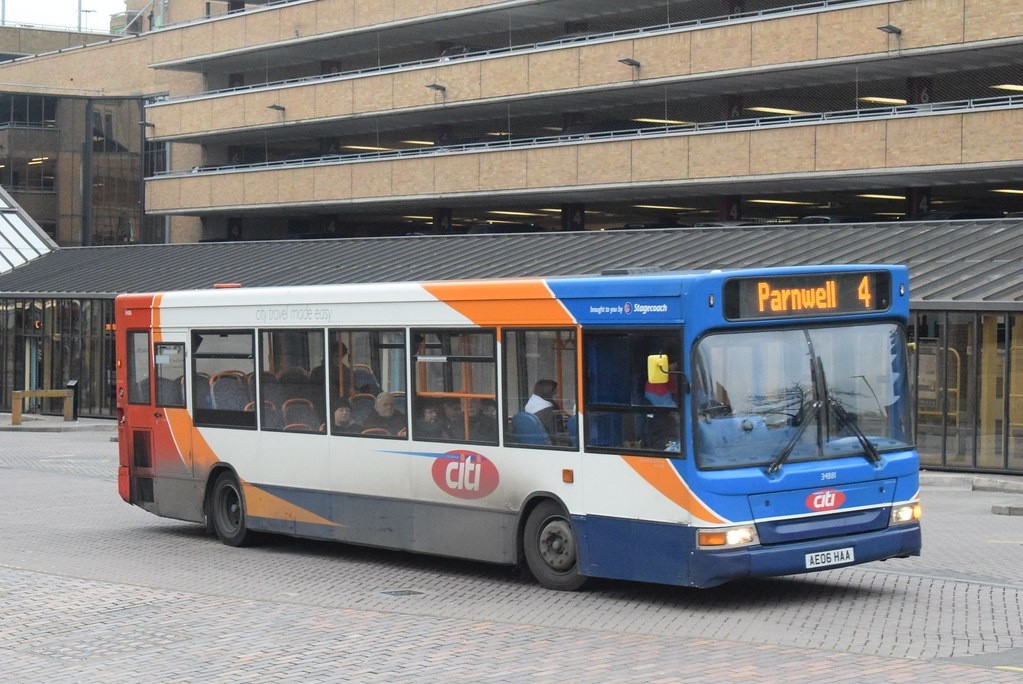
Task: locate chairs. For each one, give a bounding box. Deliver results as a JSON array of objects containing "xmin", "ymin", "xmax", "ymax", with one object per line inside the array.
[{"xmin": 138, "ymin": 364, "xmax": 597, "ymax": 448}]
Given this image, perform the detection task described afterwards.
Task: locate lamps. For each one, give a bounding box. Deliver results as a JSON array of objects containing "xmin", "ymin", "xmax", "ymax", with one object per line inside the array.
[
  {"xmin": 138, "ymin": 121, "xmax": 153, "ymax": 128},
  {"xmin": 267, "ymin": 105, "xmax": 284, "ymax": 111},
  {"xmin": 877, "ymin": 25, "xmax": 901, "ymax": 34},
  {"xmin": 617, "ymin": 58, "xmax": 640, "ymax": 68},
  {"xmin": 425, "ymin": 84, "xmax": 445, "ymax": 90}
]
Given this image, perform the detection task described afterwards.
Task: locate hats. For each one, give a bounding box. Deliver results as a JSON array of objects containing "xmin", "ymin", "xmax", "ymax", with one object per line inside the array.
[{"xmin": 332, "ymin": 396, "xmax": 352, "ymax": 414}]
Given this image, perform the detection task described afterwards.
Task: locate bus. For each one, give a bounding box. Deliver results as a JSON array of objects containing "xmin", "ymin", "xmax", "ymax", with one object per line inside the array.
[{"xmin": 113, "ymin": 263, "xmax": 922, "ymax": 593}]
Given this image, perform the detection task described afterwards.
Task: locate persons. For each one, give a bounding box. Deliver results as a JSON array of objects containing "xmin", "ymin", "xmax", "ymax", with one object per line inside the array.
[
  {"xmin": 525, "ymin": 380, "xmax": 568, "ymax": 446},
  {"xmin": 312, "ymin": 341, "xmax": 499, "ymax": 439}
]
